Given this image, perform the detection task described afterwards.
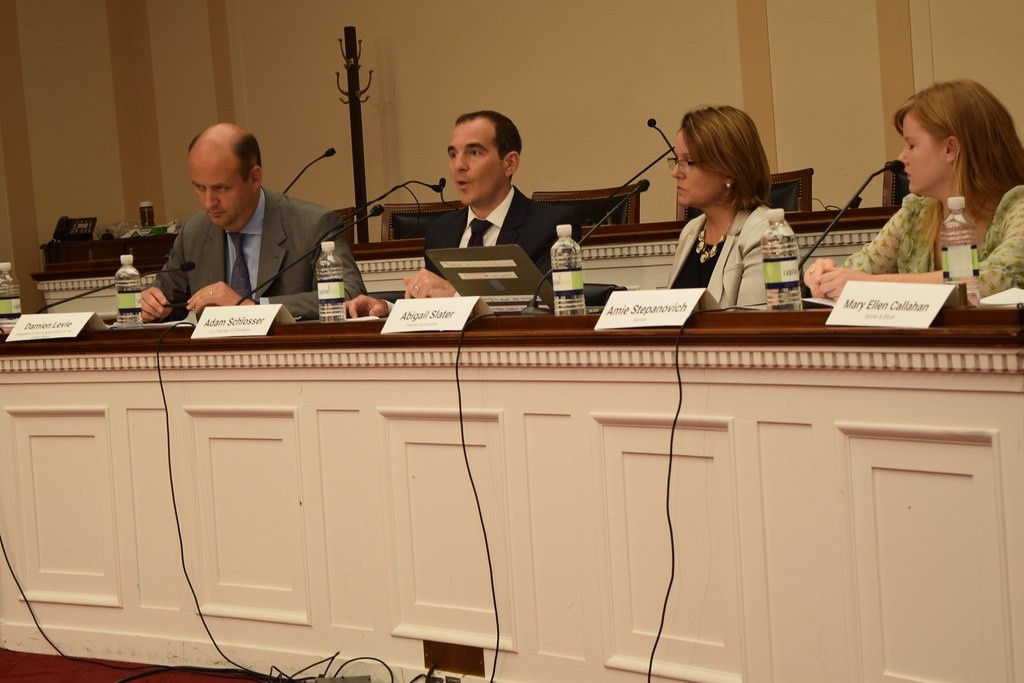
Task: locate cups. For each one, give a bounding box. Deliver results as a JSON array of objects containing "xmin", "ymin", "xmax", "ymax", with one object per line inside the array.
[{"xmin": 139, "ymin": 201, "xmax": 155, "ymax": 227}]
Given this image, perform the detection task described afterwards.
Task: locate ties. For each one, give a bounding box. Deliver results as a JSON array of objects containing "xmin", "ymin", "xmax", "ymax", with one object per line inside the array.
[
  {"xmin": 466, "ymin": 218, "xmax": 492, "ymax": 247},
  {"xmin": 230, "ymin": 232, "xmax": 252, "ymax": 300}
]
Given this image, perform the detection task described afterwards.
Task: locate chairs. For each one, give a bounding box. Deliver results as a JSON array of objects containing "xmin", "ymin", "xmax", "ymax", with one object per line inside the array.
[{"xmin": 330, "ymin": 162, "xmax": 920, "ymax": 241}]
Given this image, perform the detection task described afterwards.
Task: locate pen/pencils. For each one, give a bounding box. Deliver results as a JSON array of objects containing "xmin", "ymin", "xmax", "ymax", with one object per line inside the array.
[{"xmin": 162, "ymin": 304, "xmax": 188, "ymax": 307}]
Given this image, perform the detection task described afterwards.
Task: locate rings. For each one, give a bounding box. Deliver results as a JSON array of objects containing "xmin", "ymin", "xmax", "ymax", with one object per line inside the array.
[
  {"xmin": 209, "ymin": 291, "xmax": 213, "ymax": 296},
  {"xmin": 414, "ymin": 285, "xmax": 420, "ymax": 291}
]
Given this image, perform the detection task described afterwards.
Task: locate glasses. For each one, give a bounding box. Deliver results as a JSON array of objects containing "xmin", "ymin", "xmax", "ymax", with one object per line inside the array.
[{"xmin": 667, "ymin": 158, "xmax": 694, "ymax": 171}]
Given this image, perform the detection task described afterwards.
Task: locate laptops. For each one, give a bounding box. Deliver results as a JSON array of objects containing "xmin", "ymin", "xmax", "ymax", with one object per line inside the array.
[{"xmin": 425, "ymin": 245, "xmax": 607, "ymax": 315}]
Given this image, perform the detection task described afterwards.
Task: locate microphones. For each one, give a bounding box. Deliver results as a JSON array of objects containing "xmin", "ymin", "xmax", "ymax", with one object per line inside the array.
[
  {"xmin": 284, "ymin": 148, "xmax": 337, "ymax": 197},
  {"xmin": 236, "ymin": 178, "xmax": 462, "ymax": 307},
  {"xmin": 522, "ymin": 118, "xmax": 677, "ymax": 315},
  {"xmin": 36, "ymin": 261, "xmax": 196, "ymax": 315},
  {"xmin": 773, "ymin": 160, "xmax": 905, "ymax": 310}
]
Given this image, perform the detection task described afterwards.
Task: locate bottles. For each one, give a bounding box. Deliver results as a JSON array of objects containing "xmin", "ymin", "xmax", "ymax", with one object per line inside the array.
[
  {"xmin": 315, "ymin": 242, "xmax": 347, "ymax": 321},
  {"xmin": 115, "ymin": 254, "xmax": 142, "ymax": 329},
  {"xmin": 0, "ymin": 262, "xmax": 21, "ymax": 334},
  {"xmin": 939, "ymin": 196, "xmax": 981, "ymax": 309},
  {"xmin": 761, "ymin": 208, "xmax": 803, "ymax": 311},
  {"xmin": 550, "ymin": 224, "xmax": 586, "ymax": 316}
]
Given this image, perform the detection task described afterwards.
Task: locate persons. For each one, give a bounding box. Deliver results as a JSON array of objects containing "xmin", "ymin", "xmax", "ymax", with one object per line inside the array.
[
  {"xmin": 340, "ymin": 108, "xmax": 561, "ymax": 317},
  {"xmin": 138, "ymin": 122, "xmax": 368, "ymax": 319},
  {"xmin": 803, "ymin": 79, "xmax": 1024, "ymax": 306},
  {"xmin": 655, "ymin": 104, "xmax": 799, "ymax": 311}
]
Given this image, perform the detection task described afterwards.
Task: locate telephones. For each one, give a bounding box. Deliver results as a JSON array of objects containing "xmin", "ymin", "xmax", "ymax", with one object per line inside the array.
[{"xmin": 53, "ymin": 216, "xmax": 97, "ymax": 242}]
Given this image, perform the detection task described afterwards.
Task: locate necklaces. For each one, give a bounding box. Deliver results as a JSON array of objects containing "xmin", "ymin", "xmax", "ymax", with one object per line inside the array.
[{"xmin": 695, "ymin": 211, "xmax": 736, "ymax": 263}]
